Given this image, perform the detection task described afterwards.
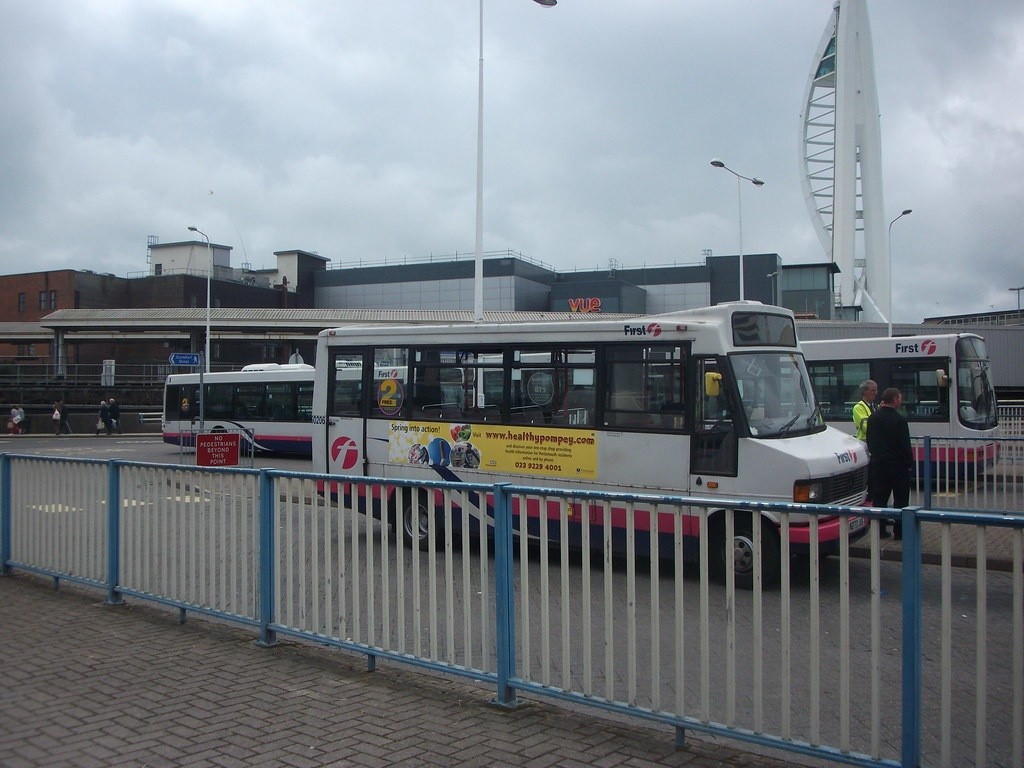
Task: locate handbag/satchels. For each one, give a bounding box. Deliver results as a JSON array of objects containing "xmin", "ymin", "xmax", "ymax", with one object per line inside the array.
[
  {"xmin": 96, "ymin": 420, "xmax": 104, "ymax": 429},
  {"xmin": 110, "ymin": 418, "xmax": 120, "ymax": 429},
  {"xmin": 53, "ymin": 409, "xmax": 60, "ymax": 419},
  {"xmin": 13, "ymin": 414, "xmax": 21, "ymax": 424},
  {"xmin": 7, "ymin": 421, "xmax": 14, "ymax": 428}
]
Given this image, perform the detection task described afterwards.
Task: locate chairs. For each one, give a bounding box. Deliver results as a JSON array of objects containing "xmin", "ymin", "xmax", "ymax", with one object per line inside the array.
[{"xmin": 371, "ymin": 399, "xmax": 595, "ymax": 425}]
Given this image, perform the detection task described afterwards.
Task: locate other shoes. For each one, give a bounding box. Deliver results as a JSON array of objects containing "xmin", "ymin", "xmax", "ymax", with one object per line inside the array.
[
  {"xmin": 880, "ymin": 531, "xmax": 891, "ymax": 539},
  {"xmin": 894, "ymin": 536, "xmax": 902, "ymax": 540}
]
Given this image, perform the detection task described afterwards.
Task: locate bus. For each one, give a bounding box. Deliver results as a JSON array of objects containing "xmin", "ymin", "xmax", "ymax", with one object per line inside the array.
[
  {"xmin": 162, "ymin": 363, "xmax": 463, "ymax": 452},
  {"xmin": 312, "ymin": 299, "xmax": 874, "ymax": 588},
  {"xmin": 646, "ymin": 334, "xmax": 1001, "ymax": 471}
]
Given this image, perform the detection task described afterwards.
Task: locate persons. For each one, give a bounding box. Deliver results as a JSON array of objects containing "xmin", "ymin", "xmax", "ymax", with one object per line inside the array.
[
  {"xmin": 853, "ymin": 379, "xmax": 878, "ymax": 441},
  {"xmin": 454, "ymin": 443, "xmax": 480, "ymax": 468},
  {"xmin": 866, "ymin": 388, "xmax": 916, "ymax": 541},
  {"xmin": 108, "ymin": 398, "xmax": 123, "ymax": 434},
  {"xmin": 53, "ymin": 399, "xmax": 73, "ymax": 435},
  {"xmin": 96, "ymin": 401, "xmax": 111, "ymax": 435},
  {"xmin": 9, "ymin": 405, "xmax": 26, "ymax": 434},
  {"xmin": 417, "ymin": 447, "xmax": 429, "ymax": 466}
]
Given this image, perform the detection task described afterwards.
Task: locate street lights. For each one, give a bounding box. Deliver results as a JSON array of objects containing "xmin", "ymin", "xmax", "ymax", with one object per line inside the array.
[
  {"xmin": 187, "ymin": 226, "xmax": 212, "ymax": 373},
  {"xmin": 709, "ymin": 157, "xmax": 766, "ymax": 301},
  {"xmin": 477, "ymin": 0, "xmax": 566, "ymax": 409},
  {"xmin": 888, "ymin": 209, "xmax": 913, "ymax": 337}
]
